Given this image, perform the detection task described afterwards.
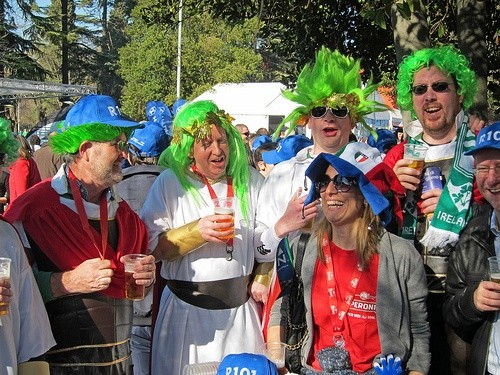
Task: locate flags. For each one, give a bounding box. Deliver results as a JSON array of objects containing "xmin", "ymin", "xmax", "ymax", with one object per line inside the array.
[{"xmin": 145, "ymin": 101, "xmax": 172, "ymax": 133}]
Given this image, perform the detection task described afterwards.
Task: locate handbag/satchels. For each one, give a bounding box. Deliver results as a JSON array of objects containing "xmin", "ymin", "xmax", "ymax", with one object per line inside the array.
[{"xmin": 284, "ymin": 232, "xmax": 309, "ymax": 373}]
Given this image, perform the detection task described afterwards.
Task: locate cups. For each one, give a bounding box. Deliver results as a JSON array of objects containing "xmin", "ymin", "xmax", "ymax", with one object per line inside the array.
[
  {"xmin": 0, "ymin": 258, "xmax": 12, "ymax": 315},
  {"xmin": 404, "ymin": 143, "xmax": 429, "ymax": 187},
  {"xmin": 211, "ymin": 197, "xmax": 237, "ymax": 239},
  {"xmin": 488, "ymin": 257, "xmax": 500, "ymax": 294},
  {"xmin": 122, "ymin": 254, "xmax": 145, "ymax": 301},
  {"xmin": 295, "ymin": 191, "xmax": 313, "ymax": 233}
]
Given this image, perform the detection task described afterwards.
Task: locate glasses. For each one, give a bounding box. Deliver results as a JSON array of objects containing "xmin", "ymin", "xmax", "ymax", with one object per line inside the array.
[
  {"xmin": 311, "ymin": 105, "xmax": 350, "ymax": 119},
  {"xmin": 411, "ymin": 80, "xmax": 456, "ymax": 94},
  {"xmin": 239, "ymin": 132, "xmax": 250, "ymax": 137},
  {"xmin": 315, "ymin": 173, "xmax": 359, "ymax": 192}
]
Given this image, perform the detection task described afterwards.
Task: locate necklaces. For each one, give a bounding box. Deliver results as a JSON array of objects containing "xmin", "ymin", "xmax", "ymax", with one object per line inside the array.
[{"xmin": 421, "ymin": 132, "xmax": 452, "ymax": 146}]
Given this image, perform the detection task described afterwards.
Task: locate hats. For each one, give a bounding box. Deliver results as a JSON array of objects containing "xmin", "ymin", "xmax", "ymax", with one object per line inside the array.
[
  {"xmin": 216, "ymin": 353, "xmax": 278, "ymax": 375},
  {"xmin": 367, "ymin": 129, "xmax": 396, "ymax": 153},
  {"xmin": 252, "ymin": 135, "xmax": 271, "ymax": 153},
  {"xmin": 58, "ymin": 93, "xmax": 146, "ymax": 135},
  {"xmin": 262, "ymin": 135, "xmax": 312, "ymax": 164},
  {"xmin": 127, "ymin": 120, "xmax": 171, "ymax": 157},
  {"xmin": 304, "ymin": 140, "xmax": 406, "ymax": 228},
  {"xmin": 464, "ymin": 122, "xmax": 500, "ymax": 155}
]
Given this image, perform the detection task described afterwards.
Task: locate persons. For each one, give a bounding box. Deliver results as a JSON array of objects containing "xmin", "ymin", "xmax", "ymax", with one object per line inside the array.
[
  {"xmin": 385, "ymin": 45, "xmax": 484, "ymax": 375},
  {"xmin": 4, "ymin": 96, "xmax": 156, "ymax": 375},
  {"xmin": 113, "ymin": 121, "xmax": 167, "ymax": 375},
  {"xmin": 236, "ymin": 124, "xmax": 313, "ymax": 178},
  {"xmin": 267, "ymin": 143, "xmax": 431, "ymax": 375},
  {"xmin": 467, "ymin": 102, "xmax": 488, "ymax": 135},
  {"xmin": 0, "ymin": 219, "xmax": 57, "ymax": 375},
  {"xmin": 253, "ymin": 92, "xmax": 385, "ymax": 343},
  {"xmin": 443, "ymin": 121, "xmax": 500, "ymax": 375},
  {"xmin": 359, "ymin": 128, "xmax": 403, "ymax": 155},
  {"xmin": 0, "ymin": 132, "xmax": 66, "ymax": 219},
  {"xmin": 141, "ymin": 99, "xmax": 275, "ymax": 375}
]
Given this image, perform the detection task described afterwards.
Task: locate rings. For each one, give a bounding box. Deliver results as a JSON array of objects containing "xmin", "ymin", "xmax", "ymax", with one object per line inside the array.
[{"xmin": 260, "ymin": 299, "xmax": 262, "ymax": 301}]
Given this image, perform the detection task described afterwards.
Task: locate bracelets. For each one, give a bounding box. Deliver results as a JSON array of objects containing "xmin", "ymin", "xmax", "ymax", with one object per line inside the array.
[{"xmin": 145, "ymin": 279, "xmax": 155, "ymax": 288}]
